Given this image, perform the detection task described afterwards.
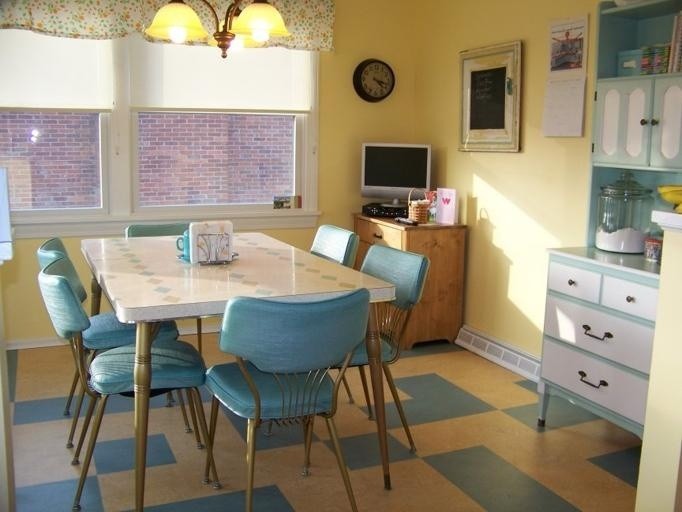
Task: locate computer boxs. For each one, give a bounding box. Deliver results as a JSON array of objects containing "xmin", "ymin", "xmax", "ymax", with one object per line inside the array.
[{"xmin": 362, "ymin": 202, "xmax": 409, "ymax": 217}]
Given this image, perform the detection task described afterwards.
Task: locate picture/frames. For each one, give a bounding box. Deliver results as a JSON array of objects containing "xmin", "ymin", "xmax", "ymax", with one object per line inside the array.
[{"xmin": 457, "ymin": 39, "xmax": 522, "ymax": 152}]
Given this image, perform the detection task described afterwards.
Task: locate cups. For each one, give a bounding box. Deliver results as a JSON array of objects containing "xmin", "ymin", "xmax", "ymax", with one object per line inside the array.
[
  {"xmin": 644, "ymin": 238, "xmax": 662, "ymax": 262},
  {"xmin": 176, "ymin": 229, "xmax": 190, "ymax": 257}
]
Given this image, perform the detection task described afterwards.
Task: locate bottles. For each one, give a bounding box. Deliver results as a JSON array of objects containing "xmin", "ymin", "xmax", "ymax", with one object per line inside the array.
[{"xmin": 594, "ymin": 171, "xmax": 654, "ymax": 254}]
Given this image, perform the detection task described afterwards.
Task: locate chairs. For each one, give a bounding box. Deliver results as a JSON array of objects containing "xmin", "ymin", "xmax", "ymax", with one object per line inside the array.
[{"xmin": 37, "ymin": 224, "xmax": 430, "ymax": 511}]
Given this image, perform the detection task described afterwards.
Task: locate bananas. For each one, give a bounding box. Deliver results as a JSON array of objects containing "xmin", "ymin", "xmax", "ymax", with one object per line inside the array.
[{"xmin": 657, "ymin": 185, "xmax": 682, "ymax": 214}]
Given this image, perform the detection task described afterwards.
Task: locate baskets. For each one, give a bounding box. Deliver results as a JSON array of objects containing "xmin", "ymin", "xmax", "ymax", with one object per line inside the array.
[{"xmin": 408, "ymin": 188, "xmax": 429, "ymax": 224}]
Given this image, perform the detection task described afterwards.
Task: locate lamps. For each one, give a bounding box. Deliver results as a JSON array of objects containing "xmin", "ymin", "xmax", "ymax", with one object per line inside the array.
[
  {"xmin": 145, "ymin": 0, "xmax": 292, "ymax": 59},
  {"xmin": 352, "ymin": 58, "xmax": 395, "ymax": 103}
]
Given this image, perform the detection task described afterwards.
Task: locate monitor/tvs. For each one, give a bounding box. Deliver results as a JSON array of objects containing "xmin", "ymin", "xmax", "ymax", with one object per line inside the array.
[{"xmin": 361, "ymin": 142, "xmax": 432, "ymax": 207}]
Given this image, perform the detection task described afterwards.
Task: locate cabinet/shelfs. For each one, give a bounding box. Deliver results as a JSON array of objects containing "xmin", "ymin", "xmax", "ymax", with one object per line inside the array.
[
  {"xmin": 350, "ymin": 213, "xmax": 468, "ymax": 351},
  {"xmin": 536, "ymin": 245, "xmax": 661, "ymax": 440},
  {"xmin": 586, "ymin": 1, "xmax": 682, "ymax": 250}
]
